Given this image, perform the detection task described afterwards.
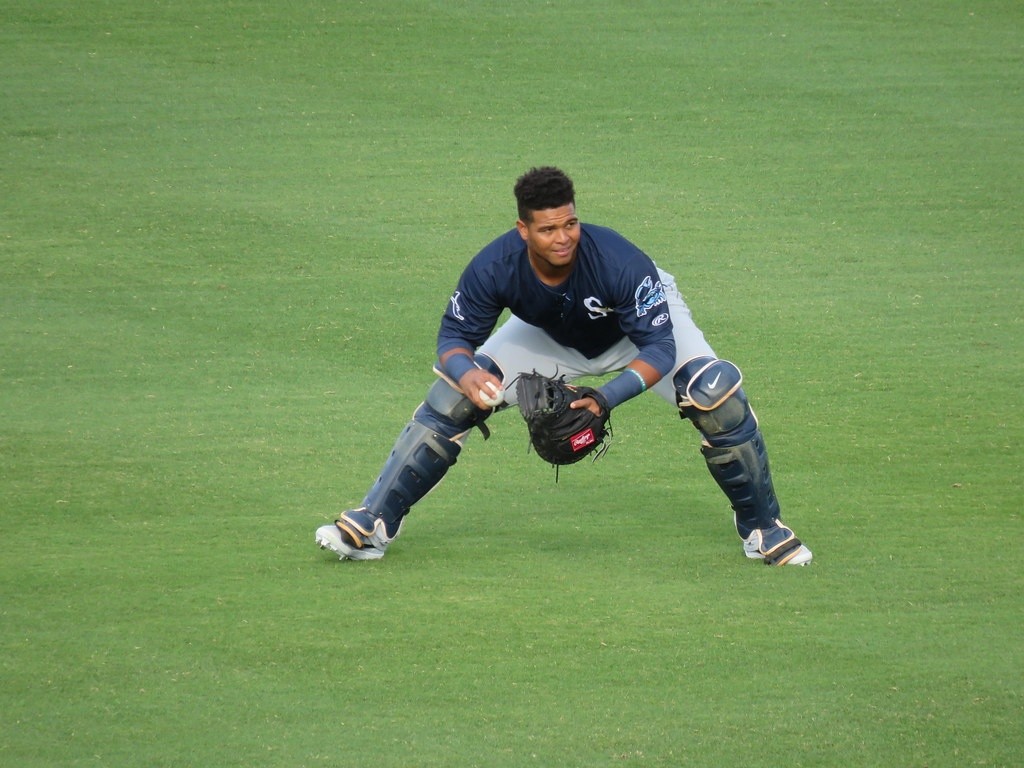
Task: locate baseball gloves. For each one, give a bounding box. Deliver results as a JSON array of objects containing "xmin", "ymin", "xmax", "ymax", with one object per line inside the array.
[{"xmin": 516, "ymin": 371, "xmax": 610, "ymax": 466}]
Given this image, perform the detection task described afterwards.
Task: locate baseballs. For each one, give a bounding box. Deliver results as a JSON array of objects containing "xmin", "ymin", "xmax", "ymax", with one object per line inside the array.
[{"xmin": 479, "ymin": 381, "xmax": 505, "ymax": 407}]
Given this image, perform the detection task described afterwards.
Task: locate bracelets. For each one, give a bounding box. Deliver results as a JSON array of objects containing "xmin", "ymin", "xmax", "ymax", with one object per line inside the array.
[
  {"xmin": 599, "ymin": 368, "xmax": 647, "ymax": 409},
  {"xmin": 445, "ymin": 353, "xmax": 476, "ymax": 384}
]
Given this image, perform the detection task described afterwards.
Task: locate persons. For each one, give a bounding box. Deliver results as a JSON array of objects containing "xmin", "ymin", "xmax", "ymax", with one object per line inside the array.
[{"xmin": 315, "ymin": 166, "xmax": 812, "ymax": 567}]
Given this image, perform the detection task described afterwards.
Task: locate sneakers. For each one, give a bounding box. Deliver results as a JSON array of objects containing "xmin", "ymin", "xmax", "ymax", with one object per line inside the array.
[
  {"xmin": 315, "ymin": 524, "xmax": 386, "ymax": 562},
  {"xmin": 742, "ymin": 530, "xmax": 812, "ymax": 567}
]
